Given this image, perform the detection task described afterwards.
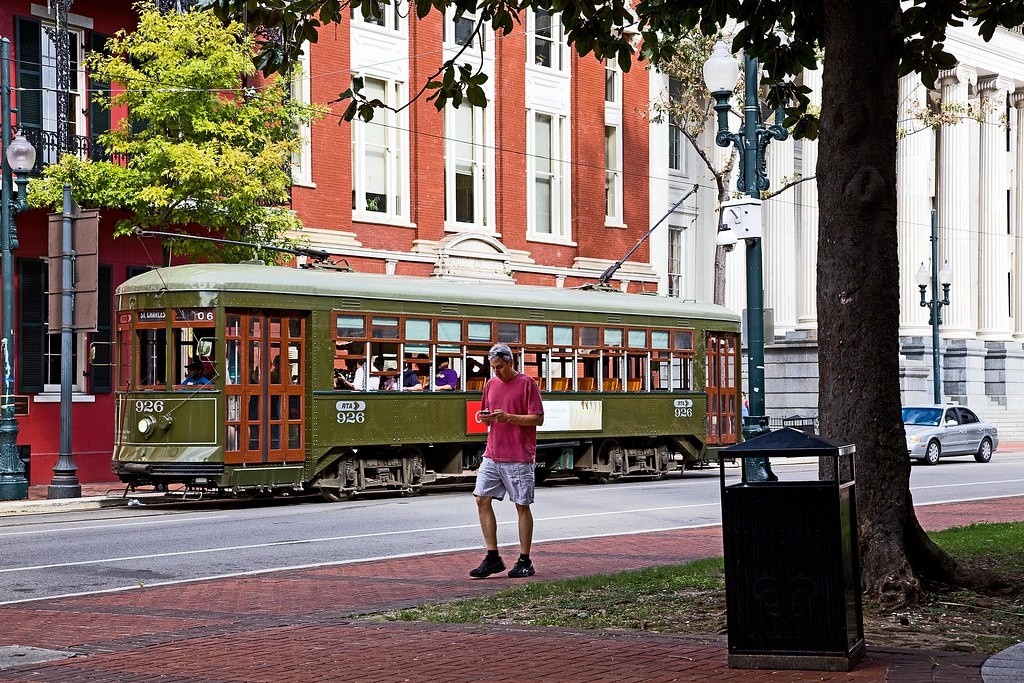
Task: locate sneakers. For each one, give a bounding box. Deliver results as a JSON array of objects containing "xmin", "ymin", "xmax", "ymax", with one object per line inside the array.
[
  {"xmin": 469, "ymin": 555, "xmax": 505, "ymax": 577},
  {"xmin": 508, "ymin": 559, "xmax": 535, "ymax": 578}
]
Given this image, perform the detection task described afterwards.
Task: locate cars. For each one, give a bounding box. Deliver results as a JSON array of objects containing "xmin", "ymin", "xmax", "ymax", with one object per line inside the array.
[{"xmin": 901, "ymin": 405, "xmax": 1000, "ymax": 467}]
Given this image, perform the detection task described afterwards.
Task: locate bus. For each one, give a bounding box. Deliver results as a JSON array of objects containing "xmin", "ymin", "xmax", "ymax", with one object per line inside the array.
[{"xmin": 98, "ymin": 183, "xmax": 743, "ymax": 511}]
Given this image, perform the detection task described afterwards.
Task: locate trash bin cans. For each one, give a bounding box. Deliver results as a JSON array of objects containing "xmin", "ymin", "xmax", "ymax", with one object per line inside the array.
[{"xmin": 716, "ymin": 427, "xmax": 867, "ymax": 669}]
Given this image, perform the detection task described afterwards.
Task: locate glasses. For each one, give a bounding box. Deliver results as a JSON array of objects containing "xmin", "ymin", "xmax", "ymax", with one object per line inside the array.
[{"xmin": 488, "ymin": 351, "xmax": 512, "ymax": 358}]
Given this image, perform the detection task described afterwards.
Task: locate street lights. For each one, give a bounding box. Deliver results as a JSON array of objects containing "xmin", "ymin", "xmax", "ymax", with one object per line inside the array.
[
  {"xmin": 0, "ymin": 37, "xmax": 36, "ymax": 500},
  {"xmin": 916, "ymin": 258, "xmax": 953, "ymax": 405},
  {"xmin": 700, "ymin": 32, "xmax": 769, "ymax": 484}
]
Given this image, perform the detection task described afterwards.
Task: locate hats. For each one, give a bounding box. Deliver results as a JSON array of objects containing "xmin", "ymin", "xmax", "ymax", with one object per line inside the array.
[{"xmin": 184, "ymin": 361, "xmax": 203, "ymax": 369}]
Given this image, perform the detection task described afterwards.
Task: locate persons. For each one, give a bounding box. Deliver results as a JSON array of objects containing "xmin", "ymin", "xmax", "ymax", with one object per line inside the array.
[
  {"xmin": 470, "ymin": 343, "xmax": 545, "ymax": 577},
  {"xmin": 333, "ymin": 335, "xmax": 488, "ymax": 391},
  {"xmin": 248, "ymin": 353, "xmax": 300, "ymax": 450},
  {"xmin": 156, "ymin": 379, "xmax": 162, "ymax": 385},
  {"xmin": 178, "ymin": 359, "xmax": 213, "ymax": 391}
]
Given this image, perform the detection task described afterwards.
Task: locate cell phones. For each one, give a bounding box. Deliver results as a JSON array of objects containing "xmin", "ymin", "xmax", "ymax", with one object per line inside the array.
[{"xmin": 481, "ymin": 411, "xmax": 490, "ymax": 414}]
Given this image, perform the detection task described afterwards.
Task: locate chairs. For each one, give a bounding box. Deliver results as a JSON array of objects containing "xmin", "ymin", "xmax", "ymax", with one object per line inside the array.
[
  {"xmin": 418, "ymin": 376, "xmax": 486, "ymax": 391},
  {"xmin": 533, "ymin": 376, "xmax": 643, "ymax": 390}
]
